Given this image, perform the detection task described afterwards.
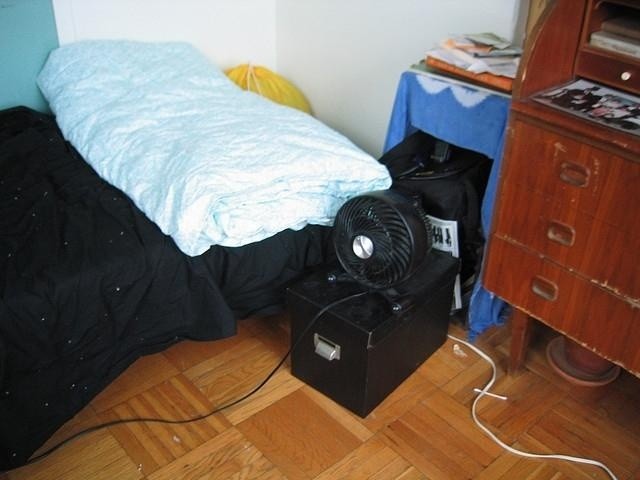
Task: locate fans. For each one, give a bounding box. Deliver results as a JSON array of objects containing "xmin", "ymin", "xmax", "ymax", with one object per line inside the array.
[{"xmin": 327, "ymin": 188, "xmax": 434, "ymax": 313}]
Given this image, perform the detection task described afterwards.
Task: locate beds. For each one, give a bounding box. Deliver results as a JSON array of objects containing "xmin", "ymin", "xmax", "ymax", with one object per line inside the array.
[{"xmin": 0, "ymin": 106, "xmax": 343, "ymax": 472}]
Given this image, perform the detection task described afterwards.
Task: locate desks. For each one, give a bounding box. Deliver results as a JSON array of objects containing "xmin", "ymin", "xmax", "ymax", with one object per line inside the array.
[{"xmin": 383, "ymin": 55, "xmax": 515, "ymax": 341}]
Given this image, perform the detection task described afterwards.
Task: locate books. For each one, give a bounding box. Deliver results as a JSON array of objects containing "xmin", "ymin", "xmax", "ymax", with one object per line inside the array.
[{"xmin": 407, "ymin": 31, "xmax": 525, "ymax": 100}]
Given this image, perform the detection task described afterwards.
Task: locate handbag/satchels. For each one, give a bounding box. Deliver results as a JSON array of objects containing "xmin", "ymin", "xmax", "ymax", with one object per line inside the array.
[{"xmin": 377, "ymin": 129, "xmax": 494, "ymax": 259}]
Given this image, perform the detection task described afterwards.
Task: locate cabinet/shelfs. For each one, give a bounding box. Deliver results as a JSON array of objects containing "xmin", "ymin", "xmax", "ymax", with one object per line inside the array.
[{"xmin": 482, "ymin": 1, "xmax": 640, "ymax": 384}]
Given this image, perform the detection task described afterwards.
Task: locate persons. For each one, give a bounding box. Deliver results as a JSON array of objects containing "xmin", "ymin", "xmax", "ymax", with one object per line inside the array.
[{"xmin": 549, "ymin": 85, "xmax": 640, "ymax": 133}]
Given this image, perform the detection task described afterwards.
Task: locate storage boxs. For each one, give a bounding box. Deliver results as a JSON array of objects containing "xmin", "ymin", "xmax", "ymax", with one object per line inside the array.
[{"xmin": 284, "ymin": 247, "xmax": 461, "ymax": 418}]
